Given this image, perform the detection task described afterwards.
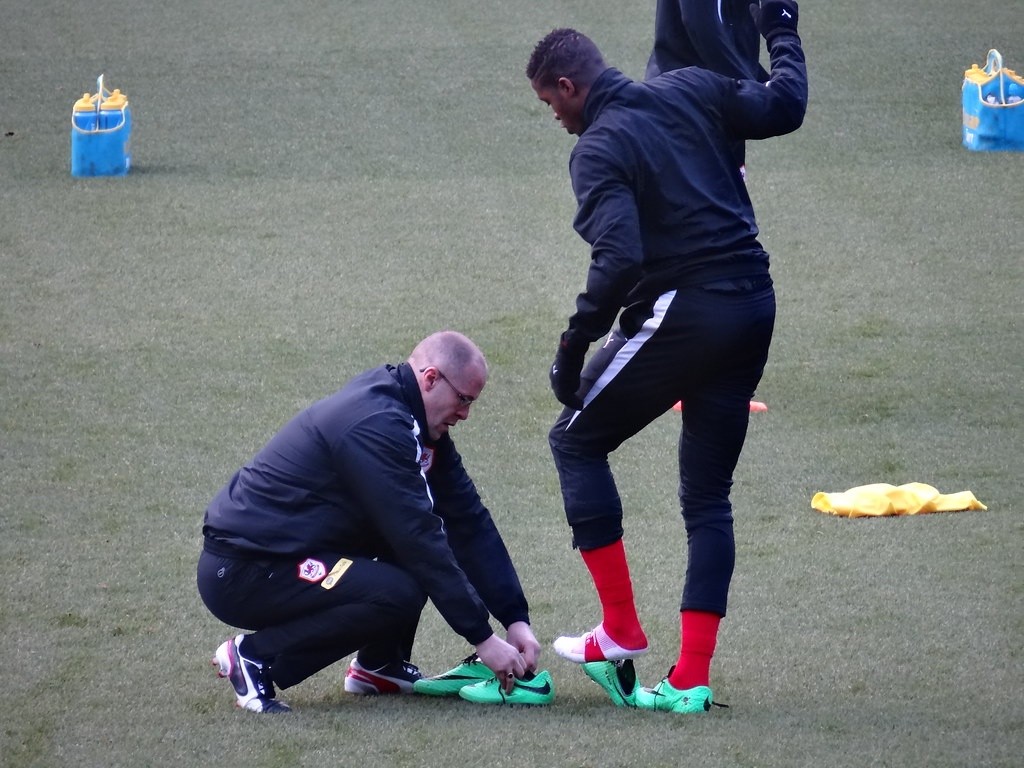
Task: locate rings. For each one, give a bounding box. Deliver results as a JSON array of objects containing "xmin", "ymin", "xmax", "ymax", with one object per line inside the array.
[{"xmin": 506, "ymin": 673, "xmax": 514, "ymax": 679}]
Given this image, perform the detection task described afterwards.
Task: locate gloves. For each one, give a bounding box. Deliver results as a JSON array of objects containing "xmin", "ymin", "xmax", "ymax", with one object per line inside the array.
[
  {"xmin": 750, "ymin": 0, "xmax": 802, "ymax": 52},
  {"xmin": 550, "ymin": 333, "xmax": 585, "ymax": 410}
]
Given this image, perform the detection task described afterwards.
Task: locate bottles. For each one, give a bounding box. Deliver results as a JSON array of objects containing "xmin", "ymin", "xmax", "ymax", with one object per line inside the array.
[
  {"xmin": 964, "ymin": 64, "xmax": 1024, "ymax": 84},
  {"xmin": 74, "ymin": 89, "xmax": 127, "ymax": 131}
]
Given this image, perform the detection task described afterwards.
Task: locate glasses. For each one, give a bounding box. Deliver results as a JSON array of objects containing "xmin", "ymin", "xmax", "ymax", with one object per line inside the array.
[{"xmin": 420, "ymin": 367, "xmax": 474, "ymax": 408}]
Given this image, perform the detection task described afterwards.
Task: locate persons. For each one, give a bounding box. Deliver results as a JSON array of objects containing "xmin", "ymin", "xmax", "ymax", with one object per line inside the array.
[
  {"xmin": 526, "ymin": 0, "xmax": 808, "ymax": 715},
  {"xmin": 197, "ymin": 331, "xmax": 540, "ymax": 712}
]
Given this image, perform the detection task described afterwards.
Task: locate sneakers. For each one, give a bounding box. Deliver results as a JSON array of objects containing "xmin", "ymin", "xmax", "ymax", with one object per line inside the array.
[
  {"xmin": 413, "ymin": 652, "xmax": 496, "ymax": 696},
  {"xmin": 582, "ymin": 659, "xmax": 640, "ymax": 709},
  {"xmin": 458, "ymin": 670, "xmax": 555, "ymax": 705},
  {"xmin": 345, "ymin": 658, "xmax": 422, "ymax": 695},
  {"xmin": 212, "ymin": 634, "xmax": 293, "ymax": 713},
  {"xmin": 635, "ymin": 665, "xmax": 712, "ymax": 713}
]
